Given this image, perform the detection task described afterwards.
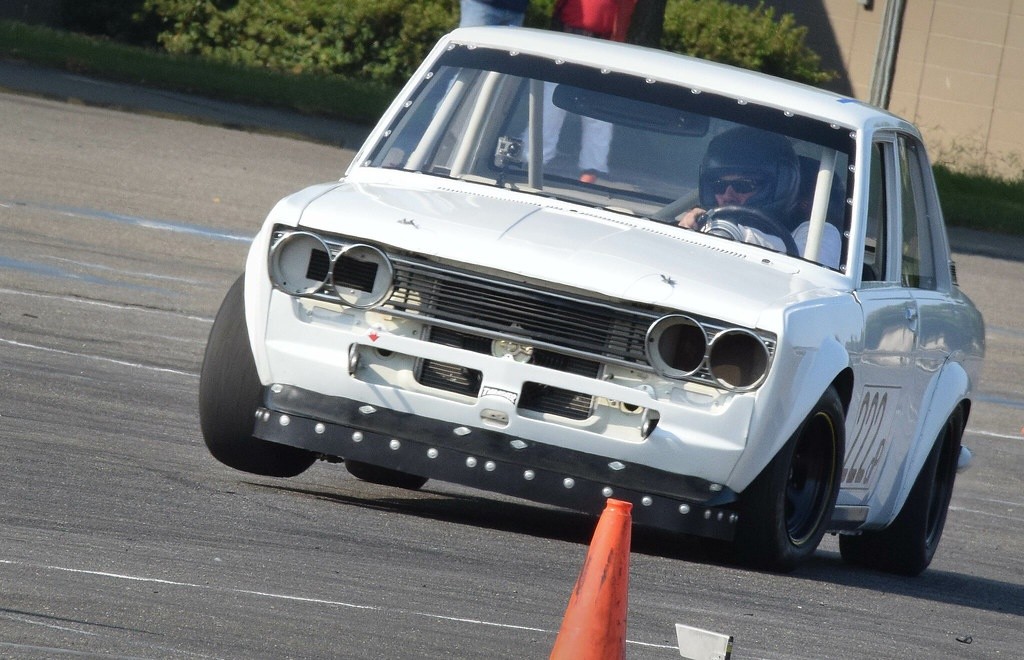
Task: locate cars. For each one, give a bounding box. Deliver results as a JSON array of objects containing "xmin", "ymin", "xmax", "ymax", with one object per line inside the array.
[{"xmin": 198, "ymin": 26, "xmax": 985, "ymax": 578}]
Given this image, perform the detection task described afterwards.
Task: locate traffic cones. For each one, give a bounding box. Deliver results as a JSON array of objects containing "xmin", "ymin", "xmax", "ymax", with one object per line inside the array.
[{"xmin": 548, "ymin": 498, "xmax": 632, "ymax": 660}]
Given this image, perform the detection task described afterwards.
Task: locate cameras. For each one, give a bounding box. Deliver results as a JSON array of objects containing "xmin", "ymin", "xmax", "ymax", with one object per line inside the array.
[{"xmin": 495, "ymin": 136, "xmax": 524, "ymax": 162}]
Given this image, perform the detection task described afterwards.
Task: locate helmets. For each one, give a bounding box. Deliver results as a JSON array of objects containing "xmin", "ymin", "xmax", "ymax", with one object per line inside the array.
[{"xmin": 696, "ymin": 127, "xmax": 802, "ymax": 215}]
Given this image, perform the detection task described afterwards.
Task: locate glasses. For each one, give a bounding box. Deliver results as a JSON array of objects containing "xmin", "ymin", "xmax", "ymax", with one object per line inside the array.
[{"xmin": 710, "ymin": 178, "xmax": 770, "ymax": 194}]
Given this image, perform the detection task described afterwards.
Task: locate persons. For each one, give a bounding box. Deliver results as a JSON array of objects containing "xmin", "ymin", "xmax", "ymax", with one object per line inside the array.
[
  {"xmin": 459, "ymin": 0, "xmax": 636, "ymax": 184},
  {"xmin": 678, "ymin": 127, "xmax": 841, "ymax": 270}
]
